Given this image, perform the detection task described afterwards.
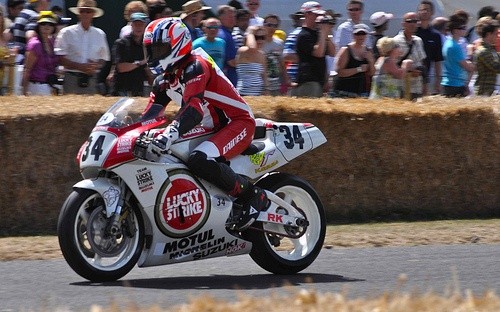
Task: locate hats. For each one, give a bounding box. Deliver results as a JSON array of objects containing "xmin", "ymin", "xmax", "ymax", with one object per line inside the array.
[
  {"xmin": 180, "ymin": 0, "xmax": 212, "ymax": 19},
  {"xmin": 352, "ymin": 24, "xmax": 370, "ymax": 33},
  {"xmin": 300, "ymin": 1, "xmax": 325, "ymax": 15},
  {"xmin": 377, "ymin": 36, "xmax": 395, "ymax": 52},
  {"xmin": 37, "ymin": 15, "xmax": 57, "ymax": 24},
  {"xmin": 131, "ymin": 13, "xmax": 149, "ymax": 22},
  {"xmin": 68, "ymin": 0, "xmax": 104, "ymax": 18},
  {"xmin": 476, "ymin": 16, "xmax": 497, "ymax": 32},
  {"xmin": 370, "ymin": 11, "xmax": 392, "ymax": 26}
]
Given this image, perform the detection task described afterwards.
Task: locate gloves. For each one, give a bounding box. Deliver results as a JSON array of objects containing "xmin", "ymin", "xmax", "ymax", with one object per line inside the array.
[{"xmin": 147, "ymin": 127, "xmax": 178, "ymax": 156}]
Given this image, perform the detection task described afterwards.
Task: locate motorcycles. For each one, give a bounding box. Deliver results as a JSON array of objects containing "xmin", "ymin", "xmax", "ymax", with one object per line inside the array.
[{"xmin": 56, "ymin": 96, "xmax": 328, "ymax": 284}]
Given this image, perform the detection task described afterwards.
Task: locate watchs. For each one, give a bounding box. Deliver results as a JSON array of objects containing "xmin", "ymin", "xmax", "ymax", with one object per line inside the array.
[{"xmin": 135, "ymin": 60, "xmax": 141, "ymax": 66}]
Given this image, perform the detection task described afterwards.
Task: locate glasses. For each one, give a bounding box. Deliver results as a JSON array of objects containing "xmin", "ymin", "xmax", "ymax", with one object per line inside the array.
[
  {"xmin": 355, "ymin": 33, "xmax": 366, "ymax": 35},
  {"xmin": 264, "ymin": 22, "xmax": 277, "ymax": 28},
  {"xmin": 207, "ymin": 26, "xmax": 217, "ymax": 29},
  {"xmin": 40, "ymin": 23, "xmax": 54, "ymax": 26},
  {"xmin": 406, "ymin": 19, "xmax": 417, "ymax": 23},
  {"xmin": 456, "ymin": 25, "xmax": 466, "ymax": 30},
  {"xmin": 347, "ymin": 8, "xmax": 360, "ymax": 11},
  {"xmin": 80, "ymin": 9, "xmax": 94, "ymax": 13},
  {"xmin": 254, "ymin": 35, "xmax": 265, "ymax": 40}
]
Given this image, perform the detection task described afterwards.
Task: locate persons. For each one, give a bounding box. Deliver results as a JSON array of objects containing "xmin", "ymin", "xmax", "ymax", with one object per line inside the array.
[
  {"xmin": 442, "ymin": 6, "xmax": 500, "ymax": 98},
  {"xmin": 366, "ymin": 11, "xmax": 394, "ymax": 59},
  {"xmin": 333, "ymin": 23, "xmax": 375, "ymax": 98},
  {"xmin": 112, "ymin": 12, "xmax": 148, "ymax": 98},
  {"xmin": 53, "ymin": 0, "xmax": 111, "ymax": 94},
  {"xmin": 120, "ymin": 0, "xmax": 212, "ymax": 42},
  {"xmin": 334, "ymin": 0, "xmax": 372, "ymax": 50},
  {"xmin": 280, "ymin": 0, "xmax": 335, "ymax": 97},
  {"xmin": 128, "ymin": 20, "xmax": 265, "ymax": 229},
  {"xmin": 368, "ymin": 37, "xmax": 413, "ymax": 100},
  {"xmin": 23, "ymin": 10, "xmax": 60, "ymax": 96},
  {"xmin": 216, "ymin": 0, "xmax": 287, "ymax": 95},
  {"xmin": 191, "ymin": 18, "xmax": 226, "ymax": 71},
  {"xmin": 0, "ymin": 0, "xmax": 62, "ymax": 93},
  {"xmin": 392, "ymin": 1, "xmax": 448, "ymax": 99}
]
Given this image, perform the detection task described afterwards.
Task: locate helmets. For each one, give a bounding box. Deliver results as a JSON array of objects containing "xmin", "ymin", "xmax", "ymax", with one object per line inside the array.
[{"xmin": 142, "ymin": 17, "xmax": 192, "ymax": 75}]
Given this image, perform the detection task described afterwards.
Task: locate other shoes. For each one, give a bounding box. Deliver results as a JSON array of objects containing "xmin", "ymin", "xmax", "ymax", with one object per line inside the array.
[{"xmin": 230, "ymin": 177, "xmax": 268, "ymax": 231}]
